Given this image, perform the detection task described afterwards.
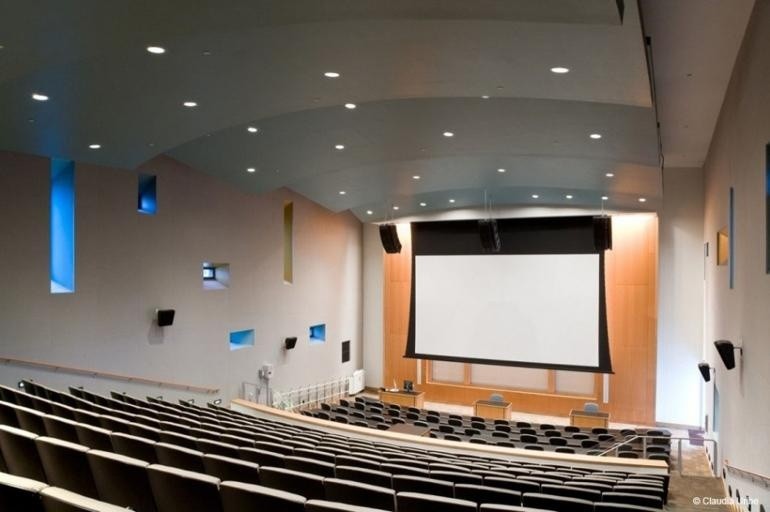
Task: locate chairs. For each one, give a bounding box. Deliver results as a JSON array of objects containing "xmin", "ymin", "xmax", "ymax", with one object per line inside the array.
[{"xmin": 0, "ymin": 378, "xmax": 672, "ymax": 512}]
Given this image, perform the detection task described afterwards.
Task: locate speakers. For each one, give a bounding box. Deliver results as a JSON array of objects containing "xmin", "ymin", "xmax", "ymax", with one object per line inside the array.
[
  {"xmin": 157, "ymin": 309, "xmax": 175, "ymax": 325},
  {"xmin": 714, "ymin": 340, "xmax": 735, "ymax": 370},
  {"xmin": 379, "ymin": 224, "xmax": 402, "ymax": 253},
  {"xmin": 285, "ymin": 337, "xmax": 297, "ymax": 349},
  {"xmin": 593, "ymin": 216, "xmax": 613, "ymax": 251},
  {"xmin": 698, "ymin": 363, "xmax": 710, "ymax": 381}
]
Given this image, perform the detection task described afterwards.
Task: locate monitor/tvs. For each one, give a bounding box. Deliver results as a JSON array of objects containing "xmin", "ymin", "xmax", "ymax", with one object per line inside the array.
[{"xmin": 403, "ymin": 380, "xmax": 413, "ymax": 392}]
